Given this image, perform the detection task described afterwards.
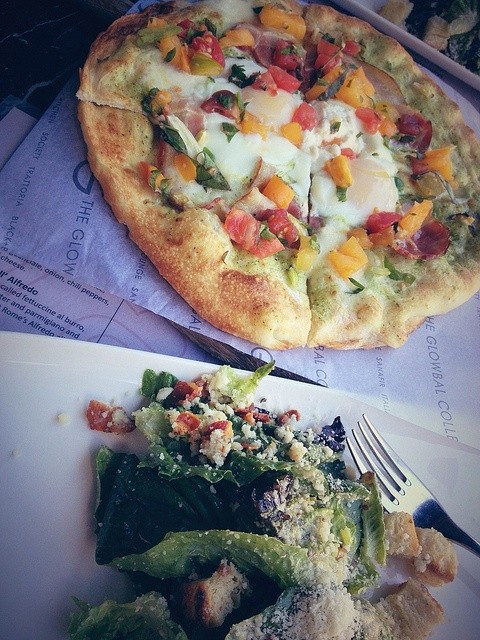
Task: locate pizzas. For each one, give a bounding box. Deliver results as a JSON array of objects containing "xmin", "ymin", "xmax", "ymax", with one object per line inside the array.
[{"xmin": 75, "ymin": 0, "xmax": 480, "ymax": 351}]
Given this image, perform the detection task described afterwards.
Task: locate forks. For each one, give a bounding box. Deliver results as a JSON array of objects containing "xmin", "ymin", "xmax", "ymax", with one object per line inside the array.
[{"xmin": 343, "ymin": 412, "xmax": 480, "ymax": 561}]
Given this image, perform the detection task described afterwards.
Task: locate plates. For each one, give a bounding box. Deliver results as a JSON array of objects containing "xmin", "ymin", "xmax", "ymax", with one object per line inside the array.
[
  {"xmin": 330, "ymin": 0, "xmax": 480, "ymax": 92},
  {"xmin": 1, "ymin": 329, "xmax": 480, "ymax": 640}
]
